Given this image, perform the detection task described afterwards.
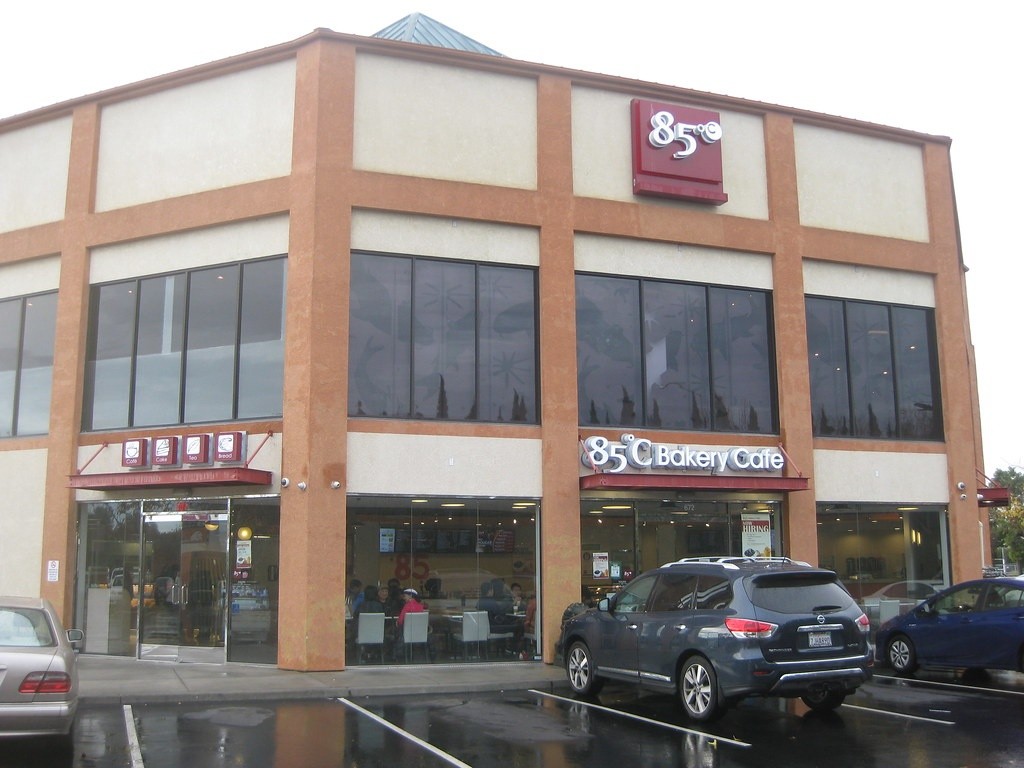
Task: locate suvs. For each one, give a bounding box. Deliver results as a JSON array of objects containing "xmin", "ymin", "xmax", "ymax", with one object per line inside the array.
[
  {"xmin": 428, "ymin": 567, "xmax": 529, "ymax": 640},
  {"xmin": 216, "ymin": 580, "xmax": 271, "ymax": 645},
  {"xmin": 561, "ymin": 554, "xmax": 874, "ymax": 722}
]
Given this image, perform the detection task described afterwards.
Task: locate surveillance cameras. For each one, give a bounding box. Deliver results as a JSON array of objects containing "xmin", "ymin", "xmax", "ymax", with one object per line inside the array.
[
  {"xmin": 958, "ymin": 482, "xmax": 965, "ymax": 490},
  {"xmin": 281, "ymin": 478, "xmax": 289, "ymax": 487},
  {"xmin": 977, "ymin": 494, "xmax": 983, "ymax": 500}
]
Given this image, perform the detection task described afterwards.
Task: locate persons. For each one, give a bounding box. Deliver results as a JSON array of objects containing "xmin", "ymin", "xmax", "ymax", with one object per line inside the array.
[
  {"xmin": 581, "ymin": 585, "xmax": 596, "ymax": 608},
  {"xmin": 345, "ymin": 579, "xmax": 442, "ymax": 663},
  {"xmin": 476, "ymin": 580, "xmax": 537, "ymax": 654}
]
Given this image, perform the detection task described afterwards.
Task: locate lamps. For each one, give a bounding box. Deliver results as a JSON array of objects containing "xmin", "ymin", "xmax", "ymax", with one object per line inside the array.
[
  {"xmin": 448, "ymin": 514, "xmax": 452, "ymax": 520},
  {"xmin": 741, "ymin": 504, "xmax": 748, "ymax": 511},
  {"xmin": 238, "ymin": 525, "xmax": 253, "ymax": 541},
  {"xmin": 433, "ymin": 514, "xmax": 439, "ymax": 522},
  {"xmin": 205, "ymin": 521, "xmax": 219, "ymax": 531},
  {"xmin": 705, "ymin": 522, "xmax": 711, "ymax": 527}
]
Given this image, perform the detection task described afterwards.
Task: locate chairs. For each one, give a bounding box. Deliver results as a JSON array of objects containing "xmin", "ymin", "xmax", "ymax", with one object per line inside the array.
[
  {"xmin": 975, "ymin": 589, "xmax": 1003, "ymax": 610},
  {"xmin": 453, "ymin": 612, "xmax": 488, "ymax": 661},
  {"xmin": 395, "ymin": 612, "xmax": 429, "ymax": 663},
  {"xmin": 482, "ymin": 611, "xmax": 514, "ymax": 660},
  {"xmin": 8, "ymin": 611, "xmax": 46, "ymax": 644},
  {"xmin": 652, "ymin": 575, "xmax": 683, "ymax": 611},
  {"xmin": 523, "ymin": 610, "xmax": 537, "ymax": 659},
  {"xmin": 355, "ymin": 613, "xmax": 385, "ymax": 665}
]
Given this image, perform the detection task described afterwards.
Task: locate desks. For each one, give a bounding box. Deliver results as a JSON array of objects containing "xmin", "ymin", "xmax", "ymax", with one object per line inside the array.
[
  {"xmin": 443, "ymin": 614, "xmax": 481, "ymax": 660},
  {"xmin": 346, "ymin": 616, "xmax": 399, "ymax": 659},
  {"xmin": 505, "ymin": 613, "xmax": 526, "ymax": 655}
]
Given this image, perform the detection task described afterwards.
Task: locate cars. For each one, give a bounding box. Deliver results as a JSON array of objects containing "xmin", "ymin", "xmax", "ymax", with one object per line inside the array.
[
  {"xmin": 861, "ymin": 579, "xmax": 944, "ymax": 628},
  {"xmin": 90, "ymin": 566, "xmax": 141, "ymax": 612},
  {"xmin": 989, "ymin": 574, "xmax": 1024, "ymax": 608},
  {"xmin": 0, "ymin": 593, "xmax": 84, "ymax": 743},
  {"xmin": 876, "ymin": 576, "xmax": 1024, "ymax": 676}
]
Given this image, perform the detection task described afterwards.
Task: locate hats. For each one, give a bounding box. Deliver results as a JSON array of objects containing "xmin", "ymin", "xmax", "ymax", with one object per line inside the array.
[
  {"xmin": 387, "ymin": 578, "xmax": 400, "ymax": 587},
  {"xmin": 379, "ymin": 583, "xmax": 390, "ymax": 590},
  {"xmin": 403, "ymin": 588, "xmax": 418, "ymax": 598}
]
